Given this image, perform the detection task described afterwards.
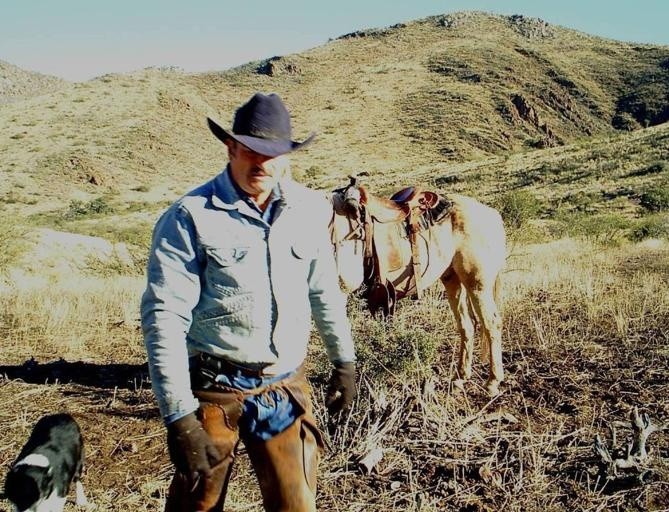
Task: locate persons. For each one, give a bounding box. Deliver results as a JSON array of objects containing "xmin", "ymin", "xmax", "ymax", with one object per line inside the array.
[{"xmin": 139, "ymin": 88, "xmax": 359, "ymax": 511}]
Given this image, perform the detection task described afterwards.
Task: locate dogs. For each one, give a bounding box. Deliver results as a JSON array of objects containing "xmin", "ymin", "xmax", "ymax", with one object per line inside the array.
[{"xmin": 0, "ymin": 412, "xmax": 90, "ymax": 512}]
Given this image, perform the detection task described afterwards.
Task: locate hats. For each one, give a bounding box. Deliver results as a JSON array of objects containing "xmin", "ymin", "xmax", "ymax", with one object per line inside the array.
[{"xmin": 207, "ymin": 93, "xmax": 318, "ymax": 156}]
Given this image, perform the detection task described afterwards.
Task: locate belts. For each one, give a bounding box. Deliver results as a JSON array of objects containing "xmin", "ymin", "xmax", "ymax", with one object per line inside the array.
[{"xmin": 201, "ymin": 353, "xmax": 273, "ymax": 377}]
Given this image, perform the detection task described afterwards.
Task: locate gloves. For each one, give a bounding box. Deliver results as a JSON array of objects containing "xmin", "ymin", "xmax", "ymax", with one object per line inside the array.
[
  {"xmin": 325, "ymin": 363, "xmax": 355, "ymax": 416},
  {"xmin": 168, "ymin": 415, "xmax": 223, "ymax": 477}
]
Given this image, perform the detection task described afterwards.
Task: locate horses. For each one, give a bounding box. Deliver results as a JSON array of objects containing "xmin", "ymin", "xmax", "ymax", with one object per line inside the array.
[{"xmin": 318, "ymin": 190, "xmax": 507, "ymax": 408}]
